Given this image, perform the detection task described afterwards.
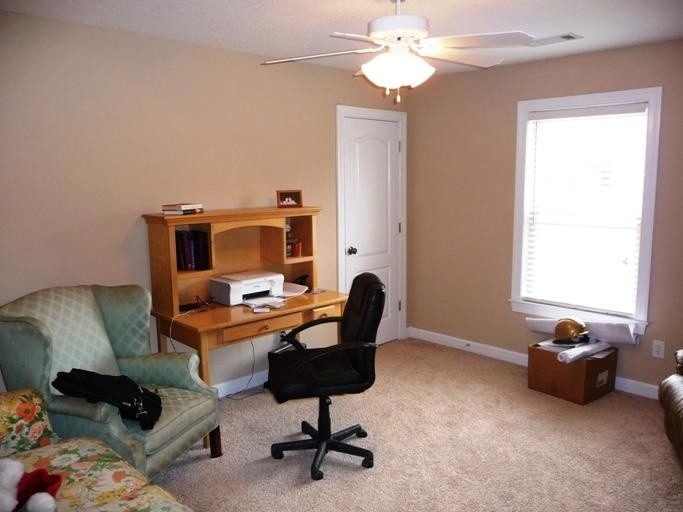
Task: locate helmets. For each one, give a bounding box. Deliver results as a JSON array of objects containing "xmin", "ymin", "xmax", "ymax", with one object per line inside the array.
[{"xmin": 555, "ymin": 318, "xmax": 589, "ymax": 340}]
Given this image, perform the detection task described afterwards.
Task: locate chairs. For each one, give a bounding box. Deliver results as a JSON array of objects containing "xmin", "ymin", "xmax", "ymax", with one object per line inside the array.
[
  {"xmin": 263, "ymin": 271, "xmax": 386, "ymax": 479},
  {"xmin": 0, "ymin": 283, "xmax": 222, "ymax": 482}
]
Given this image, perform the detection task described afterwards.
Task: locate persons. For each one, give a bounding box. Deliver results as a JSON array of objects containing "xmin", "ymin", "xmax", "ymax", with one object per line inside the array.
[
  {"xmin": 281, "ymin": 197, "xmax": 292, "ymax": 205},
  {"xmin": 288, "ymin": 197, "xmax": 297, "ymax": 204}
]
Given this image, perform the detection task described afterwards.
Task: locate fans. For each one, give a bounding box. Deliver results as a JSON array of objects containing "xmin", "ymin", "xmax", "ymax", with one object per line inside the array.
[{"xmin": 258, "ymin": 0, "xmax": 534, "ymax": 74}]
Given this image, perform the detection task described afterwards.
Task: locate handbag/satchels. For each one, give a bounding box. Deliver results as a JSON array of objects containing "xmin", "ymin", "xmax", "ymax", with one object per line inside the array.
[{"xmin": 51, "ymin": 367, "xmax": 163, "ymax": 431}]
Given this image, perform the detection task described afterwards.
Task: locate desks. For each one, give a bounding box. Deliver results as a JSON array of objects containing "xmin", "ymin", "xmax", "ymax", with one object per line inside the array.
[{"xmin": 139, "ymin": 207, "xmax": 347, "ymax": 450}]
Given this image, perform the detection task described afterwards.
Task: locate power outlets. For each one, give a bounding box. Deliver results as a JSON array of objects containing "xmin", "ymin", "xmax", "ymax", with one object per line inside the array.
[{"xmin": 651, "ymin": 340, "xmax": 664, "ymax": 358}]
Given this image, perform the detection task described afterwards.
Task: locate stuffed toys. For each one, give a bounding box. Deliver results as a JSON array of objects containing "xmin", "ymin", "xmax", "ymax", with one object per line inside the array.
[{"xmin": 0, "ymin": 456, "xmax": 63, "ymax": 512}]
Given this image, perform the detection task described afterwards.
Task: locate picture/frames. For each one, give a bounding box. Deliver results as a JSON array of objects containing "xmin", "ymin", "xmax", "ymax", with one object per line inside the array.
[{"xmin": 275, "ymin": 190, "xmax": 302, "ymax": 208}]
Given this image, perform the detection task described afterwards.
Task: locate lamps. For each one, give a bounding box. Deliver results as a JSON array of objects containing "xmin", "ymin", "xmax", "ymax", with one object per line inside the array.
[{"xmin": 360, "ymin": 40, "xmax": 436, "ymax": 104}]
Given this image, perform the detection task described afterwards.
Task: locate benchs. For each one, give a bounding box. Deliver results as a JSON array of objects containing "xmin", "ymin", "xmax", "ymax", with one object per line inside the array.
[{"xmin": 0, "ymin": 389, "xmax": 191, "ymax": 512}]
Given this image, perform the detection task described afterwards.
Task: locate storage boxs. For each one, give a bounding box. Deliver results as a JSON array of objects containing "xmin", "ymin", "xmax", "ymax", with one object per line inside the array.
[{"xmin": 527, "ymin": 341, "xmax": 618, "ymax": 405}]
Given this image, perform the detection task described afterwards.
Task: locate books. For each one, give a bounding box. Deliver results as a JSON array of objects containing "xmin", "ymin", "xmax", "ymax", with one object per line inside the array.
[
  {"xmin": 173, "ymin": 227, "xmax": 212, "ymax": 272},
  {"xmin": 160, "ymin": 202, "xmax": 203, "ymax": 211},
  {"xmin": 285, "ymin": 222, "xmax": 304, "ymax": 258},
  {"xmin": 281, "ymin": 280, "xmax": 309, "ymax": 298},
  {"xmin": 160, "ymin": 208, "xmax": 204, "ymax": 215}
]
